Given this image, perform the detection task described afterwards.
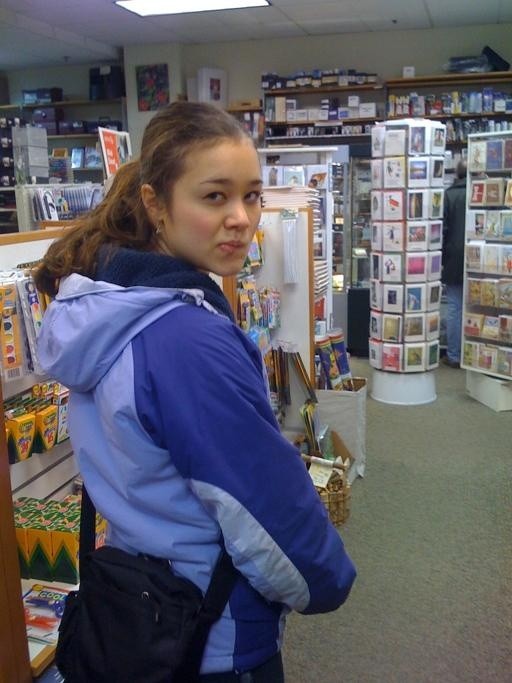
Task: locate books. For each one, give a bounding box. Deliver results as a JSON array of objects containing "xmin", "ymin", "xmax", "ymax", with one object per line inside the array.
[
  {"xmin": 368, "ymin": 125, "xmax": 445, "ymax": 373},
  {"xmin": 460, "ymin": 137, "xmax": 512, "ymax": 380}
]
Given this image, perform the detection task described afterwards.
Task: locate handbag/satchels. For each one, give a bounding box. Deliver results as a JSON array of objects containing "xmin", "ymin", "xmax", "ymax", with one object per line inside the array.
[{"xmin": 52, "ymin": 544, "xmax": 210, "ymax": 683}]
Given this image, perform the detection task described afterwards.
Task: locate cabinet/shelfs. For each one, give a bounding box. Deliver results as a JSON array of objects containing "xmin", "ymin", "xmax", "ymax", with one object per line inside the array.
[
  {"xmin": 0, "ymin": 97, "xmax": 122, "ymax": 234},
  {"xmin": 262, "ymin": 70, "xmax": 512, "ymax": 412}
]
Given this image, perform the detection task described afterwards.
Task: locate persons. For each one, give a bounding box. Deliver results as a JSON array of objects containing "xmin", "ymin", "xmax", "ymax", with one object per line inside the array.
[
  {"xmin": 441, "ymin": 158, "xmax": 466, "ymax": 368},
  {"xmin": 30, "ymin": 98, "xmax": 356, "ymax": 682}
]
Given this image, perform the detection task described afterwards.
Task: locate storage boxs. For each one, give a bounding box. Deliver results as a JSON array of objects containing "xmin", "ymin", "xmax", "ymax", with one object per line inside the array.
[{"xmin": 313, "ymin": 375, "xmax": 370, "ymax": 485}]
